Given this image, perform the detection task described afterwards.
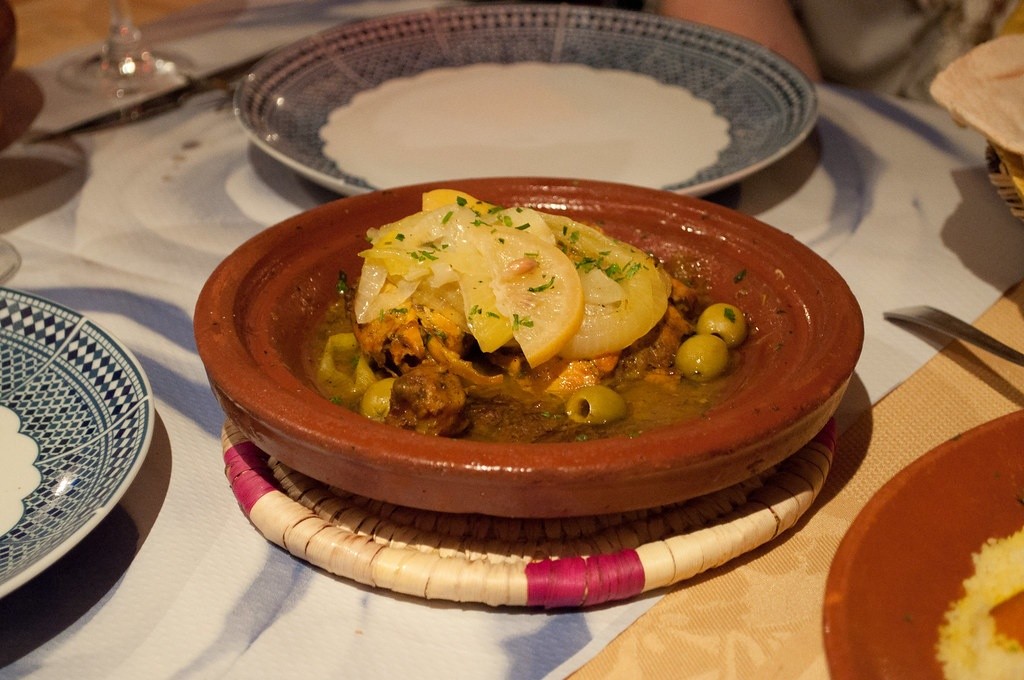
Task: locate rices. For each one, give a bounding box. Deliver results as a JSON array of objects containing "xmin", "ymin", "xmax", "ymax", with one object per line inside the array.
[{"xmin": 933, "ymin": 528, "xmax": 1023, "ymax": 680}]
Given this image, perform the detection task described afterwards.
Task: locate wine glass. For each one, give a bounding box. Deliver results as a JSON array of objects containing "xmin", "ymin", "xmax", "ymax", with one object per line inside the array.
[{"xmin": 56, "ymin": 0, "xmax": 178, "ymax": 94}]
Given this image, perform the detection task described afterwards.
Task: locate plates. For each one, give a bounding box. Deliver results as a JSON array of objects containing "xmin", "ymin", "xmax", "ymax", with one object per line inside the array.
[
  {"xmin": 191, "ymin": 178, "xmax": 859, "ymax": 515},
  {"xmin": 233, "ymin": 2, "xmax": 820, "ymax": 197},
  {"xmin": 822, "ymin": 410, "xmax": 1023, "ymax": 679},
  {"xmin": 0, "ymin": 285, "xmax": 155, "ymax": 601}
]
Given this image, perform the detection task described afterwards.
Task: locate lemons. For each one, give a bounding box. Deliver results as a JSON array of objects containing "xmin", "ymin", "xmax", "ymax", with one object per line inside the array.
[{"xmin": 356, "ymin": 185, "xmax": 670, "ymax": 370}]
